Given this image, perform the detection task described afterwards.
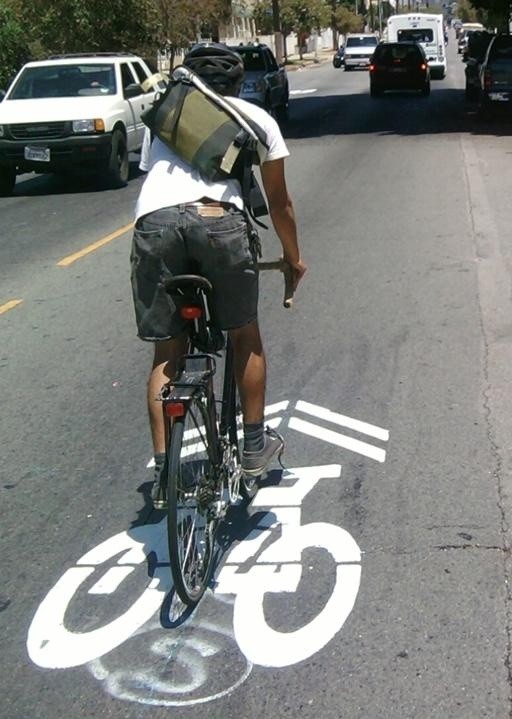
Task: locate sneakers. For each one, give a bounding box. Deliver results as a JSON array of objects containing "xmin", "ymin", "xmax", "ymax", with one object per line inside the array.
[
  {"xmin": 241, "ymin": 425, "xmax": 286, "ymax": 477},
  {"xmin": 151, "ymin": 482, "xmax": 168, "ymax": 510}
]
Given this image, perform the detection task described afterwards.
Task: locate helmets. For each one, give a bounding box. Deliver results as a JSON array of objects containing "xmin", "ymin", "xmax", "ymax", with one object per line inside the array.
[{"xmin": 183, "ymin": 44, "xmax": 244, "ymax": 97}]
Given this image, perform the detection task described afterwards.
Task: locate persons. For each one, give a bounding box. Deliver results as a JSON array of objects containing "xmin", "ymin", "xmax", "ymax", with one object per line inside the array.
[{"xmin": 129, "ymin": 40, "xmax": 310, "ymax": 510}]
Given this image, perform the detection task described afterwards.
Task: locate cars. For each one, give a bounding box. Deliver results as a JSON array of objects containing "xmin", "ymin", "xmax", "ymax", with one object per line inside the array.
[
  {"xmin": 332, "ymin": 43, "xmax": 345, "ymax": 69},
  {"xmin": 452, "ymin": 17, "xmax": 512, "ymax": 126}
]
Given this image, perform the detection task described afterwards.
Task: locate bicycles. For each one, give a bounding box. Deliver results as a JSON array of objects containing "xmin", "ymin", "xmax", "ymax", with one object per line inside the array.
[{"xmin": 148, "ymin": 249, "xmax": 296, "ymax": 608}]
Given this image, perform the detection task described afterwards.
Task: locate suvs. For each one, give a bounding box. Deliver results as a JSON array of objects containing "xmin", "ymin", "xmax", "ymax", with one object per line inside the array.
[
  {"xmin": 0, "ymin": 53, "xmax": 170, "ymax": 197},
  {"xmin": 364, "ymin": 38, "xmax": 435, "ymax": 98},
  {"xmin": 226, "ymin": 40, "xmax": 290, "ymax": 129}
]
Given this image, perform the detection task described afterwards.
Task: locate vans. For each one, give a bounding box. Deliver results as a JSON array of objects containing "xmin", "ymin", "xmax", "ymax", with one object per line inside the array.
[
  {"xmin": 379, "ymin": 12, "xmax": 449, "ymax": 82},
  {"xmin": 341, "ymin": 31, "xmax": 382, "ymax": 72}
]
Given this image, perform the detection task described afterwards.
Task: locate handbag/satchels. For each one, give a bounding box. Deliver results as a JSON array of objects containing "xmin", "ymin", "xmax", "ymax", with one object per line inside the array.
[{"xmin": 140, "ymin": 64, "xmax": 269, "ymax": 182}]
